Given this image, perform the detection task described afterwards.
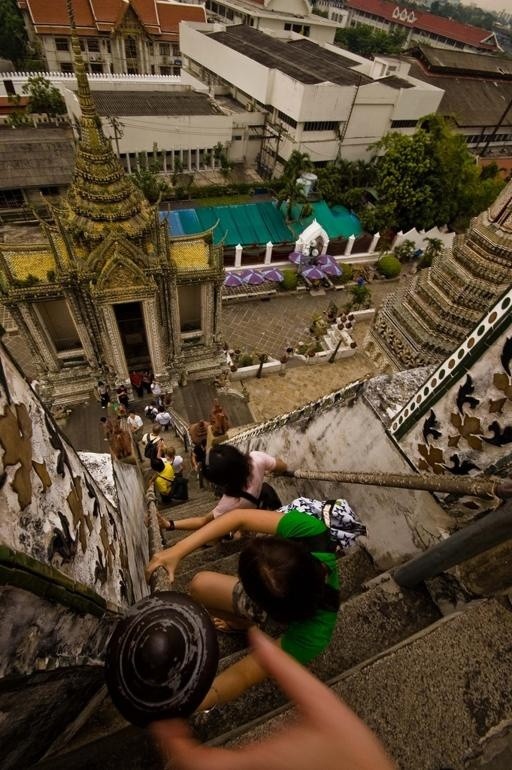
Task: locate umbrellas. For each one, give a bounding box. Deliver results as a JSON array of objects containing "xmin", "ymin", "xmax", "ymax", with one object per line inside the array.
[
  {"xmin": 241, "ymin": 268, "xmax": 266, "ymax": 294},
  {"xmin": 298, "ymin": 265, "xmax": 325, "ymax": 285},
  {"xmin": 261, "ymin": 266, "xmax": 285, "ymax": 290},
  {"xmin": 312, "ymin": 254, "xmax": 336, "ymax": 266},
  {"xmin": 316, "ymin": 263, "xmax": 342, "ymax": 276},
  {"xmin": 288, "ymin": 252, "xmax": 307, "ymax": 272},
  {"xmin": 221, "ymin": 271, "xmax": 243, "ymax": 295}
]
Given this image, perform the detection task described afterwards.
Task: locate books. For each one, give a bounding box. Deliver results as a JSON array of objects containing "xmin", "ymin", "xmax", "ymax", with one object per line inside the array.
[{"xmin": 97, "ymin": 369, "xmax": 211, "ymax": 505}]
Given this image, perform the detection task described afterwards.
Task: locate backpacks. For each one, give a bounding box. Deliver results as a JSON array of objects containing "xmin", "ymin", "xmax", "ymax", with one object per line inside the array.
[
  {"xmin": 145, "ymin": 433, "xmax": 164, "ymax": 459},
  {"xmin": 146, "ymin": 406, "xmax": 156, "ymax": 419}
]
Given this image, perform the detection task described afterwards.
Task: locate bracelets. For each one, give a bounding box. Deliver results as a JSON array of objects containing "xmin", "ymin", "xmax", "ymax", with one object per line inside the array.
[
  {"xmin": 166, "ymin": 520, "xmax": 175, "ymax": 532},
  {"xmin": 202, "ymin": 686, "xmax": 221, "ymax": 715}
]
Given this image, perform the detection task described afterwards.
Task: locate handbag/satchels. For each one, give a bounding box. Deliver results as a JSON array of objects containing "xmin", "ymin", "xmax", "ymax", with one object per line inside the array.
[
  {"xmin": 271, "ymin": 497, "xmax": 367, "ymax": 552},
  {"xmin": 258, "ymin": 481, "xmax": 283, "ymax": 511},
  {"xmin": 169, "ymin": 477, "xmax": 189, "ymax": 500},
  {"xmin": 133, "ymin": 430, "xmax": 143, "ymax": 441}
]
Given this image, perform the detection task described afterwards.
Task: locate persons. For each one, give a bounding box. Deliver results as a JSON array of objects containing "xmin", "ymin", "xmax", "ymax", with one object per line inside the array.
[
  {"xmin": 144, "ymin": 507, "xmax": 342, "ymax": 715},
  {"xmin": 151, "ymin": 623, "xmax": 401, "ymax": 769},
  {"xmin": 154, "ymin": 442, "xmax": 289, "ymax": 548}
]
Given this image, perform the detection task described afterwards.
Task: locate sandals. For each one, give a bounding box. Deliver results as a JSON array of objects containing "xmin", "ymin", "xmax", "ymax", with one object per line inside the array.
[{"xmin": 211, "ymin": 616, "xmax": 261, "ymax": 634}]
